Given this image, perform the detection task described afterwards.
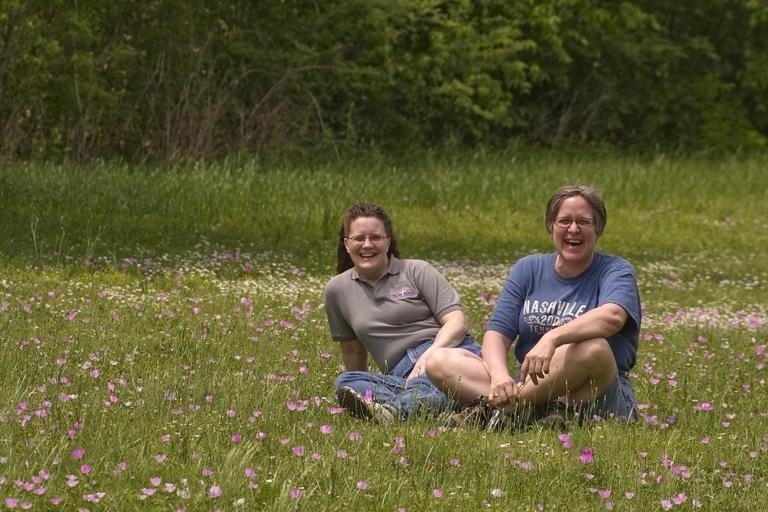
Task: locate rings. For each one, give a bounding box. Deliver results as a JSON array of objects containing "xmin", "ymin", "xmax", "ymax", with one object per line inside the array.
[{"xmin": 494, "ymin": 395, "xmax": 498, "ymax": 398}]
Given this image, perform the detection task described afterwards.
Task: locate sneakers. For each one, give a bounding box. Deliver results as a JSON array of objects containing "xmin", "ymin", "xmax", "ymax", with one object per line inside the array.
[
  {"xmin": 449, "ymin": 400, "xmax": 574, "ymax": 437},
  {"xmin": 335, "ymin": 386, "xmax": 399, "ymax": 429}
]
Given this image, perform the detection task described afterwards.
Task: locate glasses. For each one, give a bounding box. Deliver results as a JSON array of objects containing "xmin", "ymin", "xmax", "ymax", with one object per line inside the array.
[
  {"xmin": 552, "ymin": 217, "xmax": 595, "ymax": 228},
  {"xmin": 347, "ymin": 234, "xmax": 389, "ymax": 242}
]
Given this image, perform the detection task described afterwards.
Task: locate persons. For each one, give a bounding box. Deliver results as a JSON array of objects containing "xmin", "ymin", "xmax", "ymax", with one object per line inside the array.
[
  {"xmin": 424, "ymin": 183, "xmax": 643, "ymax": 433},
  {"xmin": 324, "ymin": 203, "xmax": 484, "ymax": 424}
]
